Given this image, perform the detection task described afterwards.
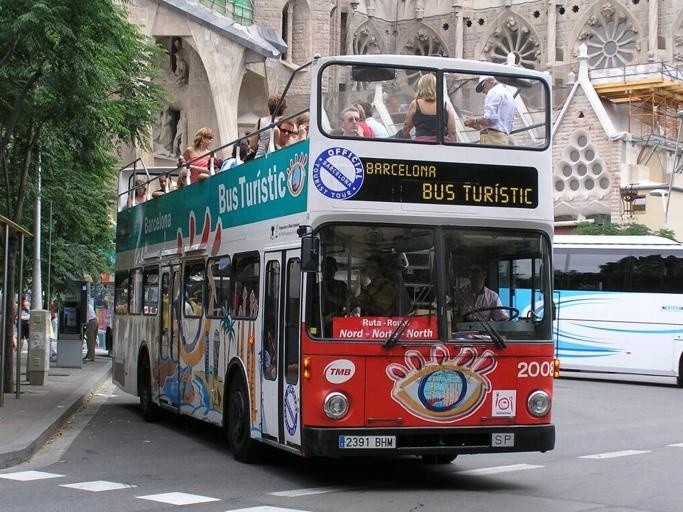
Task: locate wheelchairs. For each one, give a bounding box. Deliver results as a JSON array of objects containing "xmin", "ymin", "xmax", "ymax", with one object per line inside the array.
[{"xmin": 82, "ymin": 323, "xmax": 89, "ymax": 360}]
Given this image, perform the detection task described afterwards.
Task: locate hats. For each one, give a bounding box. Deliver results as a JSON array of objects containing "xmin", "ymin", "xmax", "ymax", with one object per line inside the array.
[{"xmin": 476, "ymin": 75, "xmax": 493, "ymax": 92}]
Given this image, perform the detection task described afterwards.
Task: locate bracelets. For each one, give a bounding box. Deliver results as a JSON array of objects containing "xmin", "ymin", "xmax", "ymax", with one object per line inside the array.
[{"xmin": 472, "ymin": 117, "xmax": 477, "ymax": 124}]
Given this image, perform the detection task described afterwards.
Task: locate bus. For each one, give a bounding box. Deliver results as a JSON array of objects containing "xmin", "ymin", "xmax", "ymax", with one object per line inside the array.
[
  {"xmin": 102, "ymin": 48, "xmax": 564, "ymax": 477},
  {"xmin": 498, "ymin": 230, "xmax": 682, "ymax": 390}
]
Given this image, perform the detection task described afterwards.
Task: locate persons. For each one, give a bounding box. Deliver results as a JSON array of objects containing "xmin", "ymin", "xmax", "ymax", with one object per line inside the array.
[
  {"xmin": 463, "ymin": 74, "xmax": 518, "ymax": 150},
  {"xmin": 83, "ymin": 305, "xmax": 99, "ymax": 362},
  {"xmin": 324, "ymin": 102, "xmax": 390, "ymax": 139},
  {"xmin": 405, "ymin": 75, "xmax": 458, "ymax": 144},
  {"xmin": 234, "ymin": 256, "xmax": 393, "ymax": 335},
  {"xmin": 15, "ymin": 292, "xmax": 31, "ymax": 353},
  {"xmin": 126, "ymin": 94, "xmax": 308, "ymax": 205},
  {"xmin": 450, "ymin": 266, "xmax": 510, "ymax": 322}
]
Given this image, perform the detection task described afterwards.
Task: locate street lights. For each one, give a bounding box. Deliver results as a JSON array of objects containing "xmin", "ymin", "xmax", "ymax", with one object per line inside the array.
[{"xmin": 648, "ymin": 110, "xmax": 682, "ymax": 227}]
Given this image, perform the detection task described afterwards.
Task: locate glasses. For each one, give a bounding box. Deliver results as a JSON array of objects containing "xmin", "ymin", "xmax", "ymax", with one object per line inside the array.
[{"xmin": 281, "ymin": 129, "xmax": 293, "ymax": 134}]
